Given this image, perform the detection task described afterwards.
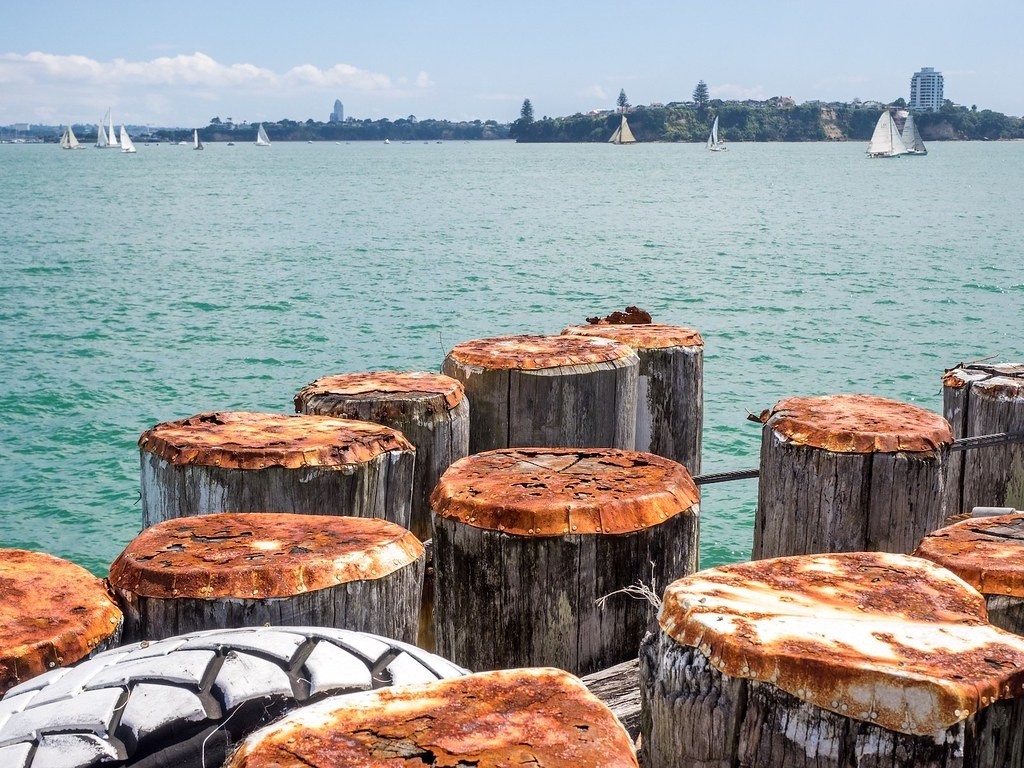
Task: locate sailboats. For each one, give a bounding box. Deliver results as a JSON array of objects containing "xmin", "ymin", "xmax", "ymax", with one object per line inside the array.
[
  {"xmin": 608, "ymin": 115, "xmax": 636, "ymax": 145},
  {"xmin": 866, "ymin": 110, "xmax": 909, "ymax": 158},
  {"xmin": 94, "ymin": 107, "xmax": 121, "ymax": 148},
  {"xmin": 193, "ymin": 129, "xmax": 203, "ymax": 150},
  {"xmin": 120, "ymin": 123, "xmax": 137, "ymax": 153},
  {"xmin": 255, "ymin": 123, "xmax": 271, "ymax": 146},
  {"xmin": 705, "ymin": 116, "xmax": 727, "ymax": 151},
  {"xmin": 60, "ymin": 121, "xmax": 86, "ymax": 149},
  {"xmin": 901, "ymin": 115, "xmax": 928, "ymax": 155}
]
